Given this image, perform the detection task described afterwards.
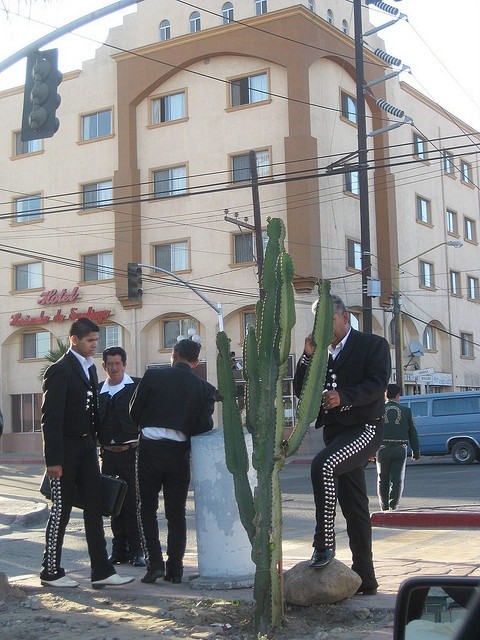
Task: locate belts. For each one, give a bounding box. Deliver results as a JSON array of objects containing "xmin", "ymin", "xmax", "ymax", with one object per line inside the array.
[{"xmin": 103, "ymin": 444, "xmax": 136, "ymax": 454}]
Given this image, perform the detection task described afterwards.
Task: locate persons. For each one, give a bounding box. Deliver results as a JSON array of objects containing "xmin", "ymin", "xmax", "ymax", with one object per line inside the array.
[
  {"xmin": 40, "ymin": 317, "xmax": 135, "ymax": 589},
  {"xmin": 96, "ymin": 346, "xmax": 147, "ymax": 567},
  {"xmin": 128, "ymin": 339, "xmax": 217, "ymax": 583},
  {"xmin": 293, "ymin": 294, "xmax": 391, "ymax": 596},
  {"xmin": 369, "ymin": 383, "xmax": 421, "ymax": 512}
]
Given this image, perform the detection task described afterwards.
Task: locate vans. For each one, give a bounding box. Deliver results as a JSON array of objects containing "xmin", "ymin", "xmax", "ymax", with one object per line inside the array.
[{"xmin": 399, "ymin": 391, "xmax": 480, "ymax": 464}]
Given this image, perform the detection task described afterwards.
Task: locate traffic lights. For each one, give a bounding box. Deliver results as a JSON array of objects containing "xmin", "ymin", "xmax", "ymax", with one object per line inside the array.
[
  {"xmin": 20, "ymin": 47, "xmax": 63, "ymax": 142},
  {"xmin": 127, "ymin": 263, "xmax": 143, "ymax": 300}
]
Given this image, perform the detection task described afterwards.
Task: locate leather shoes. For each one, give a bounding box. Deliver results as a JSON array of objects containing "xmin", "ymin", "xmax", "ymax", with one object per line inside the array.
[
  {"xmin": 165, "ymin": 572, "xmax": 181, "ymax": 583},
  {"xmin": 92, "ymin": 575, "xmax": 135, "ymax": 588},
  {"xmin": 107, "ymin": 555, "xmax": 129, "ymax": 564},
  {"xmin": 141, "ymin": 568, "xmax": 165, "ymax": 583},
  {"xmin": 133, "ymin": 555, "xmax": 146, "ymax": 566},
  {"xmin": 41, "ymin": 576, "xmax": 80, "ymax": 588},
  {"xmin": 354, "ymin": 581, "xmax": 377, "ymax": 595},
  {"xmin": 309, "ymin": 548, "xmax": 335, "ymax": 568}
]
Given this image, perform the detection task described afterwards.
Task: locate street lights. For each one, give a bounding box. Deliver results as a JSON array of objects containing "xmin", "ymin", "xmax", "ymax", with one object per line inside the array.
[{"xmin": 399, "ymin": 240, "xmax": 462, "ymax": 267}]
